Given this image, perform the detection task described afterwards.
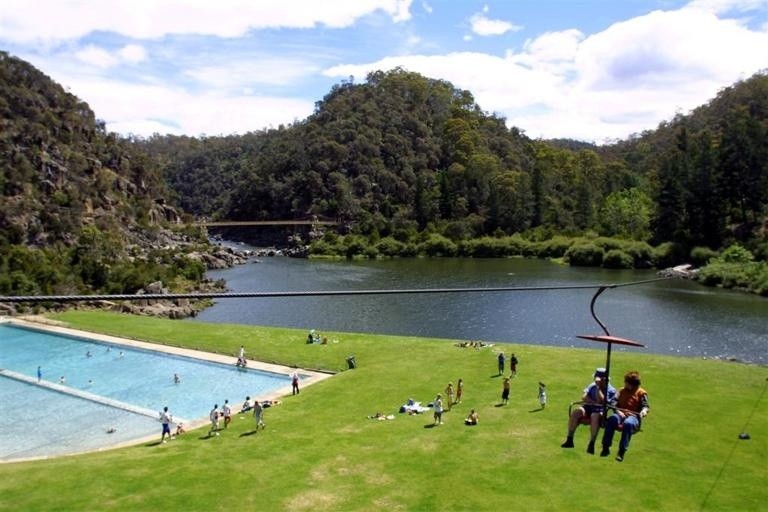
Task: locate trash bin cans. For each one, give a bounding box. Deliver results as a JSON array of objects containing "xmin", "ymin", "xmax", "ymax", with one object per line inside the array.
[{"xmin": 347, "ymin": 356, "xmax": 356, "ymax": 369}]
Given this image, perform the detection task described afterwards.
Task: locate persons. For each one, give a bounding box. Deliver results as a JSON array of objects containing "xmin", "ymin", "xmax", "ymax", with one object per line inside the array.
[
  {"xmin": 498, "ymin": 352, "xmax": 505, "ymax": 376},
  {"xmin": 560, "ymin": 368, "xmax": 616, "ymax": 454},
  {"xmin": 433, "ymin": 393, "xmax": 444, "ymax": 425},
  {"xmin": 600, "ymin": 371, "xmax": 650, "ymax": 461},
  {"xmin": 501, "ymin": 377, "xmax": 511, "ymax": 405},
  {"xmin": 537, "ymin": 382, "xmax": 546, "ymax": 410},
  {"xmin": 36, "ymin": 332, "xmax": 329, "ymax": 443},
  {"xmin": 444, "ymin": 382, "xmax": 454, "ymax": 411},
  {"xmin": 465, "ymin": 409, "xmax": 479, "ymax": 425},
  {"xmin": 510, "ymin": 353, "xmax": 518, "ymax": 375},
  {"xmin": 454, "ymin": 378, "xmax": 463, "ymax": 404}
]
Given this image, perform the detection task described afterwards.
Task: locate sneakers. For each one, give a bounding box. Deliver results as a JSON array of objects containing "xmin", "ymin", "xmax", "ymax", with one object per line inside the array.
[
  {"xmin": 615, "ymin": 448, "xmax": 627, "ymax": 462},
  {"xmin": 560, "ymin": 436, "xmax": 574, "ymax": 448},
  {"xmin": 587, "ymin": 442, "xmax": 595, "ymax": 454},
  {"xmin": 600, "ymin": 445, "xmax": 610, "ymax": 457}
]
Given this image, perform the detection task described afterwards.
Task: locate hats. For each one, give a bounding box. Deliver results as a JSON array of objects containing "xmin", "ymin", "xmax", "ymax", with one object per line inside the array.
[{"xmin": 593, "ymin": 368, "xmax": 610, "ymax": 382}]
